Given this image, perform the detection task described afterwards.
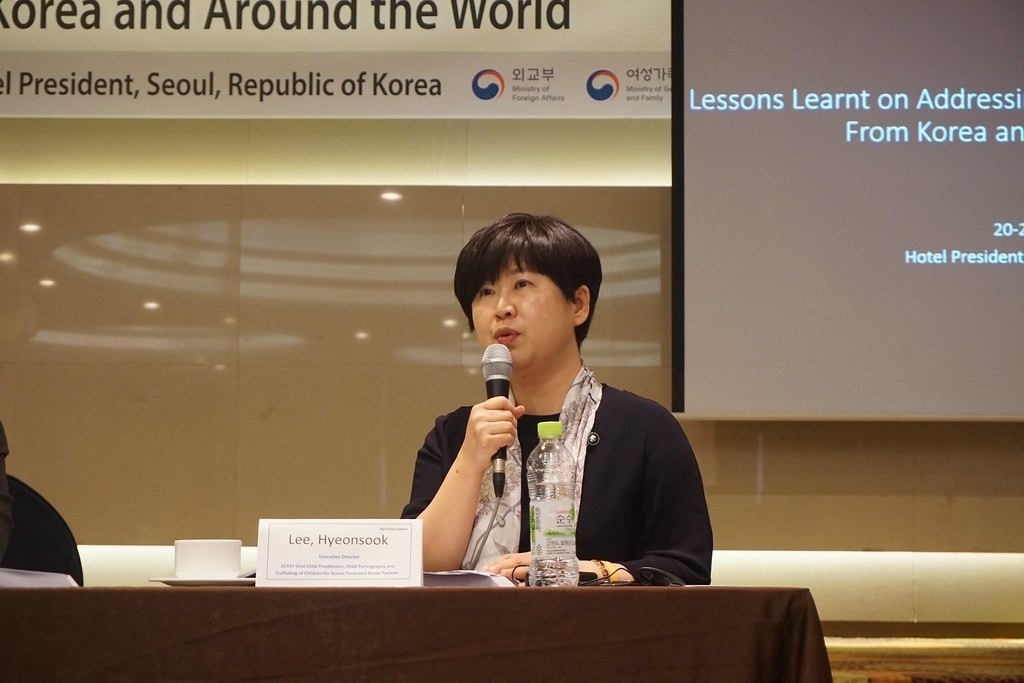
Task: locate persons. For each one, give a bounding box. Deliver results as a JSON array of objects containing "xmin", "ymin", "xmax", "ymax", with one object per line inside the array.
[{"xmin": 400, "ymin": 211, "xmax": 713, "ymax": 588}]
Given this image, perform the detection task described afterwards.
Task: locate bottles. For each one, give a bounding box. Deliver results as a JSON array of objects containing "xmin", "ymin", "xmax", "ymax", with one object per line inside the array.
[{"xmin": 529, "ymin": 419, "xmax": 579, "ymax": 585}]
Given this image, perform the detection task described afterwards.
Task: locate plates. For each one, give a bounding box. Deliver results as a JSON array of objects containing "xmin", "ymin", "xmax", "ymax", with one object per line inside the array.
[{"xmin": 148, "ymin": 574, "xmax": 256, "ymax": 585}]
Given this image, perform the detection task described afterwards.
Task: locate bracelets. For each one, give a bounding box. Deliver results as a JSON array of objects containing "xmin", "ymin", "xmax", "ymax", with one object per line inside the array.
[{"xmin": 591, "ymin": 559, "xmax": 620, "ymax": 583}]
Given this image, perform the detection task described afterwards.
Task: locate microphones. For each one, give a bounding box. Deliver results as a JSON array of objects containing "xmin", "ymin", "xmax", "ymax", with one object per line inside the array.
[{"xmin": 481, "ymin": 344, "xmax": 515, "ymax": 499}]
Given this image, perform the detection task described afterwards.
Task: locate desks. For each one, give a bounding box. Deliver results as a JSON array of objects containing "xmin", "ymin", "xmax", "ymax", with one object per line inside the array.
[{"xmin": 2, "ymin": 585, "xmax": 828, "ymax": 682}]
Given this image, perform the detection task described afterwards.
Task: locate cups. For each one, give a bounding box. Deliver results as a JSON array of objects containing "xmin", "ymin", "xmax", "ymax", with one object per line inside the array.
[{"xmin": 171, "ymin": 538, "xmax": 243, "ymax": 579}]
